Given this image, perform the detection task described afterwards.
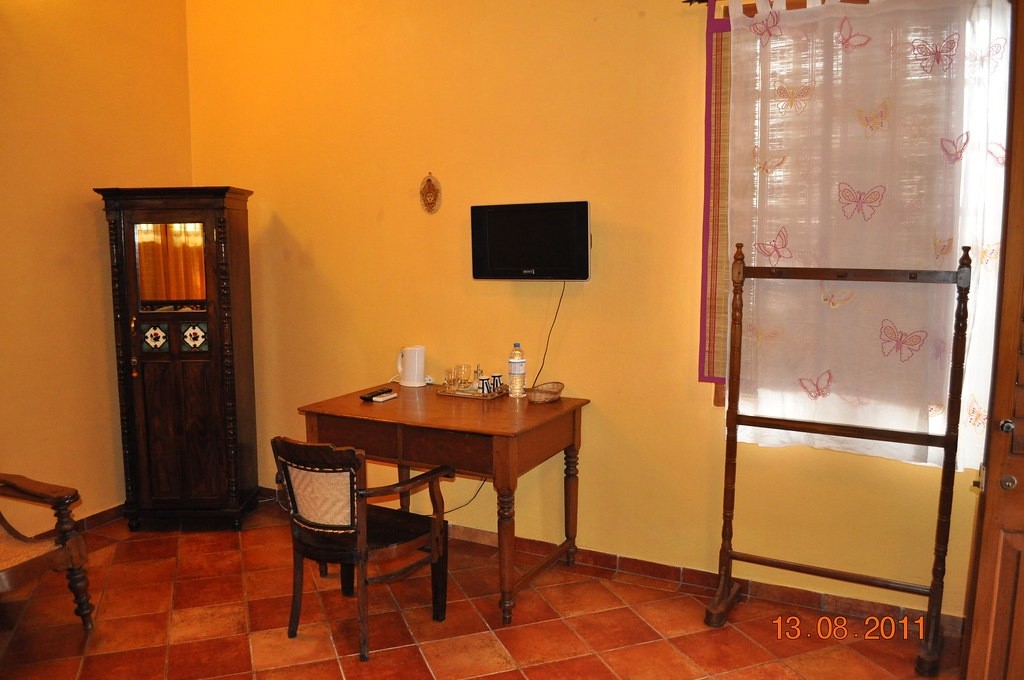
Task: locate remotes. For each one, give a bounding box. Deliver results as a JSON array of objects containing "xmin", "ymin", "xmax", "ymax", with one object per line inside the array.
[
  {"xmin": 373, "ymin": 393, "xmax": 397, "ymax": 401},
  {"xmin": 359, "ymin": 387, "xmax": 393, "ymax": 401}
]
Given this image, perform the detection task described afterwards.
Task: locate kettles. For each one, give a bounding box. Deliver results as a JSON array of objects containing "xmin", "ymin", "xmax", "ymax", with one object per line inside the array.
[{"xmin": 397, "ymin": 346, "xmax": 426, "ymax": 387}]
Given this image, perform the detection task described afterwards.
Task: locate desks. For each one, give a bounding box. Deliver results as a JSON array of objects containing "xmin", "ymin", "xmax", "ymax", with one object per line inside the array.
[{"xmin": 296, "ymin": 381, "xmax": 592, "ymax": 624}]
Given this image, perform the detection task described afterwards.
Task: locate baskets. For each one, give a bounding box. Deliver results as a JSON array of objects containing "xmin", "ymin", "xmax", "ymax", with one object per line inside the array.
[{"xmin": 523, "ymin": 382, "xmax": 564, "ymax": 404}]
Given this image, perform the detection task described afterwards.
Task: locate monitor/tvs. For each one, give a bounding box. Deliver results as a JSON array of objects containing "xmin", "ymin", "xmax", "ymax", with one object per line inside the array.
[{"xmin": 470, "ymin": 201, "xmax": 590, "ymax": 280}]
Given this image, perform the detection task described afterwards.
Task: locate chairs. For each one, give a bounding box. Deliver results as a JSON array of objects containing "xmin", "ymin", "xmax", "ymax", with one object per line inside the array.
[
  {"xmin": 271, "ymin": 436, "xmax": 457, "ymax": 662},
  {"xmin": 0, "ymin": 473, "xmax": 95, "ymax": 633}
]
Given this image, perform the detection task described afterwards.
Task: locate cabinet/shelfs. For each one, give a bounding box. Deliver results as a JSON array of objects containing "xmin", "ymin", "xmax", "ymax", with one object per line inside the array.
[{"xmin": 92, "ymin": 186, "xmax": 260, "ymax": 531}]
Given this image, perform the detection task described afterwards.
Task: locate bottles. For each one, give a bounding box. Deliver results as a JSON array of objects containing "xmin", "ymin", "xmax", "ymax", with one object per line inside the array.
[{"xmin": 508, "ymin": 343, "xmax": 527, "ymax": 398}]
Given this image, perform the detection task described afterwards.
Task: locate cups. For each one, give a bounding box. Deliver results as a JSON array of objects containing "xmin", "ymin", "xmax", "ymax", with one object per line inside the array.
[
  {"xmin": 456, "ymin": 364, "xmax": 471, "ymax": 391},
  {"xmin": 477, "ymin": 376, "xmax": 491, "ymax": 394},
  {"xmin": 491, "ymin": 374, "xmax": 503, "ymax": 392},
  {"xmin": 444, "ymin": 368, "xmax": 458, "ymax": 393}
]
